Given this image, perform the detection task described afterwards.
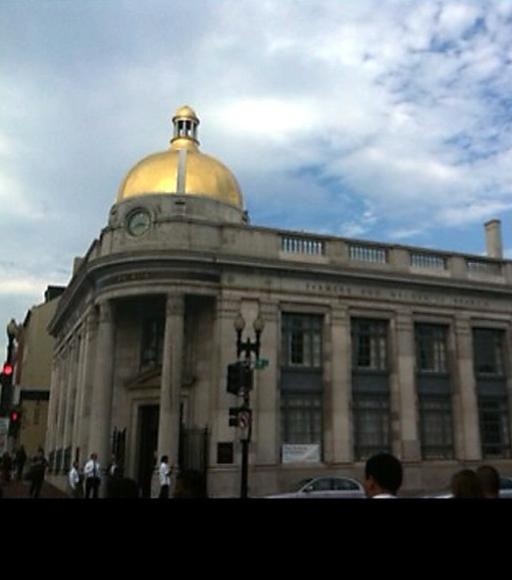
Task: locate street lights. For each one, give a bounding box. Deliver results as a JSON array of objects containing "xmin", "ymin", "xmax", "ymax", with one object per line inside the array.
[
  {"xmin": 5, "ymin": 317, "xmax": 20, "ymax": 364},
  {"xmin": 233, "ymin": 311, "xmax": 265, "ymax": 498}
]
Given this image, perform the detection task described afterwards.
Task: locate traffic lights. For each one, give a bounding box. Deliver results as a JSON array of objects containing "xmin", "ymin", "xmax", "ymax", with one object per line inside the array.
[
  {"xmin": 0, "ymin": 363, "xmax": 13, "ymax": 419},
  {"xmin": 7, "ymin": 409, "xmax": 22, "ymax": 436}
]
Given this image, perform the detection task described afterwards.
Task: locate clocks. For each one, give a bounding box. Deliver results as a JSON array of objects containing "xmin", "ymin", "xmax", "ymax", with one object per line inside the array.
[{"xmin": 122, "ymin": 208, "xmax": 156, "ymax": 238}]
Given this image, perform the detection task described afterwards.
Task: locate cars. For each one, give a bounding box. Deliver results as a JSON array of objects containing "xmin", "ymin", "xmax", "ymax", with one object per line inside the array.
[{"xmin": 250, "ymin": 474, "xmax": 367, "ymax": 498}]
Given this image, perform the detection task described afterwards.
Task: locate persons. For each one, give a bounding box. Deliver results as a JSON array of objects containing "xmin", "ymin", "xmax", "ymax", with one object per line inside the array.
[
  {"xmin": 14, "ymin": 445, "xmax": 27, "ymax": 489},
  {"xmin": 448, "ymin": 468, "xmax": 485, "ymax": 498},
  {"xmin": 104, "ymin": 454, "xmax": 120, "ymax": 498},
  {"xmin": 83, "ymin": 452, "xmax": 102, "ymax": 499},
  {"xmin": 28, "ymin": 447, "xmax": 52, "ymax": 499},
  {"xmin": 67, "ymin": 459, "xmax": 80, "ymax": 499},
  {"xmin": 156, "ymin": 455, "xmax": 174, "ymax": 499},
  {"xmin": 475, "ymin": 465, "xmax": 501, "ymax": 498},
  {"xmin": 362, "ymin": 452, "xmax": 402, "ymax": 499}
]
